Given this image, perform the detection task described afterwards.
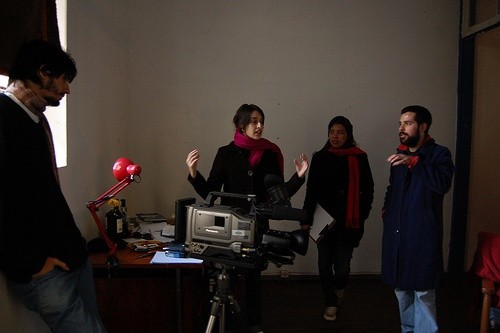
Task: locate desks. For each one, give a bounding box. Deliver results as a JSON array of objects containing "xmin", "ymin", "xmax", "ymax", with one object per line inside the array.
[{"xmin": 90, "ymin": 221, "xmax": 204, "ymax": 333}]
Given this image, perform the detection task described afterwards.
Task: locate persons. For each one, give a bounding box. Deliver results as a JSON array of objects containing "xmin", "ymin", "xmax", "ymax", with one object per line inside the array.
[
  {"xmin": 383, "ymin": 106, "xmax": 455, "ymax": 333},
  {"xmin": 0, "ymin": 35, "xmax": 107, "ymax": 333},
  {"xmin": 186, "ymin": 99, "xmax": 309, "ymax": 316},
  {"xmin": 300, "ymin": 113, "xmax": 375, "ymax": 322}
]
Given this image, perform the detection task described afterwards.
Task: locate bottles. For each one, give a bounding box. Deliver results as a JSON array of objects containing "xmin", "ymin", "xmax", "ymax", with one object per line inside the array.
[{"xmin": 104, "ymin": 199, "xmax": 134, "ymax": 243}]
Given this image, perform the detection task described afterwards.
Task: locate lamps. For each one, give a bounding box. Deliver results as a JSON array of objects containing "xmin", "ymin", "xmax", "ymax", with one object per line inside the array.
[{"xmin": 86, "ymin": 157, "xmax": 142, "ymax": 278}]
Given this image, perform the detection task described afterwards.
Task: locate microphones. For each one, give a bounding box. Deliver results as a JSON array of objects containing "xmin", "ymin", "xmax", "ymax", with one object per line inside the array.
[{"xmin": 273, "ymin": 206, "xmax": 307, "ymax": 221}]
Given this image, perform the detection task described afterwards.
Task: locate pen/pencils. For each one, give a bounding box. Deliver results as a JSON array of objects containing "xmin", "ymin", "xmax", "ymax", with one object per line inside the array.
[{"xmin": 136, "ymin": 250, "xmax": 159, "ymax": 259}]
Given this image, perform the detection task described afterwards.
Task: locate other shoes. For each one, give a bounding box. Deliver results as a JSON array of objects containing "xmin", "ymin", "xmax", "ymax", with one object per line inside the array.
[
  {"xmin": 333, "ymin": 288, "xmax": 345, "ymax": 305},
  {"xmin": 323, "ymin": 306, "xmax": 338, "ymax": 321}
]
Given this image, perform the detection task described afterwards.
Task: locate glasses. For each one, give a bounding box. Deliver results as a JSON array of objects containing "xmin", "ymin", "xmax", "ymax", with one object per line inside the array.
[{"xmin": 132, "ymin": 231, "xmax": 155, "ymax": 240}]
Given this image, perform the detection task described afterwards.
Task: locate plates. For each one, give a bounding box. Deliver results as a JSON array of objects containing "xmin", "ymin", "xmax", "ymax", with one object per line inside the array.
[{"xmin": 133, "ymin": 239, "xmax": 163, "ymax": 249}]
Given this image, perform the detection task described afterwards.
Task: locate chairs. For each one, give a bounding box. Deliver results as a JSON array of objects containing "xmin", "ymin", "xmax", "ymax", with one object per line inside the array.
[{"xmin": 469, "ymin": 230, "xmax": 500, "ymax": 333}]
